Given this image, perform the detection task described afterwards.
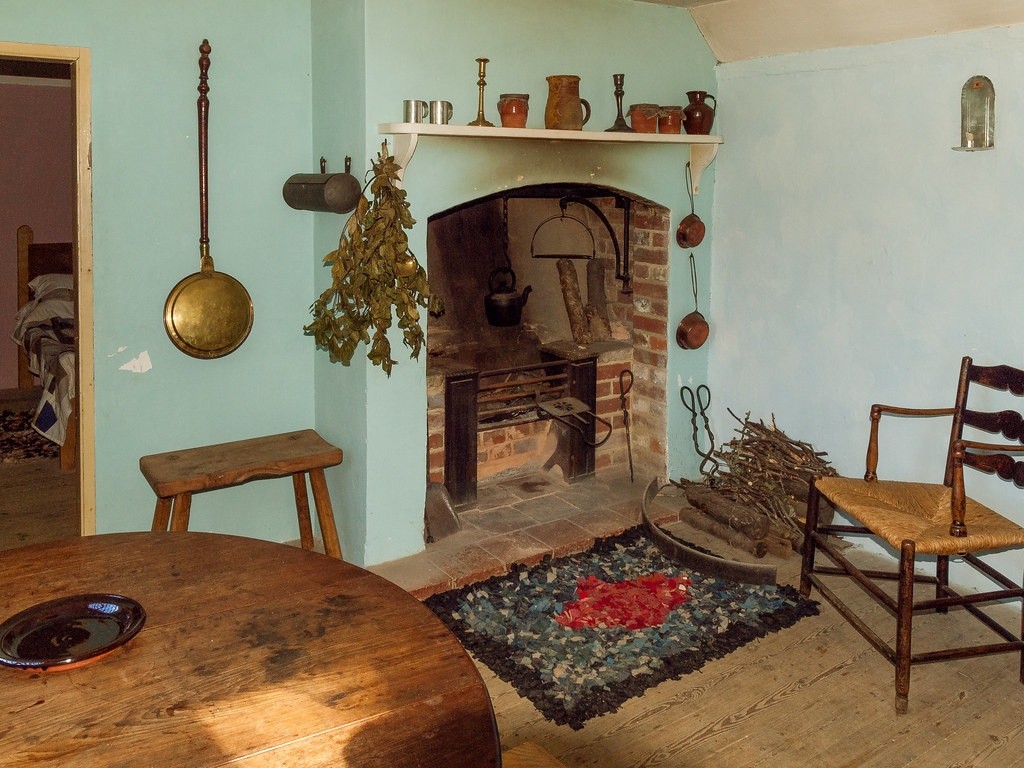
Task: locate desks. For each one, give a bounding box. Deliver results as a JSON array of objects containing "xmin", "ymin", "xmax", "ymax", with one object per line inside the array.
[{"xmin": 0, "ymin": 530, "xmax": 505, "ymax": 768}]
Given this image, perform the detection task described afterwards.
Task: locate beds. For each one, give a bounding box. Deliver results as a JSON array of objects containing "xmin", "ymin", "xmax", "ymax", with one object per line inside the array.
[{"xmin": 16, "ymin": 223, "xmax": 79, "ymax": 474}]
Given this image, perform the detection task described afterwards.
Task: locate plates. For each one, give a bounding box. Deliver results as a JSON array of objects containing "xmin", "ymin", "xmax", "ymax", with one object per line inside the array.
[{"xmin": 0, "ymin": 593, "xmax": 147, "ymax": 674}]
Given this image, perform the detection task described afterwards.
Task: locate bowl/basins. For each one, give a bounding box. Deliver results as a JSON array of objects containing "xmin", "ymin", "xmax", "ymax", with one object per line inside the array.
[
  {"xmin": 676, "ymin": 312, "xmax": 710, "ymax": 350},
  {"xmin": 676, "ymin": 214, "xmax": 705, "ymax": 249}
]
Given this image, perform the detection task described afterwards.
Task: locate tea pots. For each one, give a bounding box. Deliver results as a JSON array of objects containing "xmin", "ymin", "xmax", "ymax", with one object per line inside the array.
[
  {"xmin": 484, "ymin": 267, "xmax": 533, "ymax": 327},
  {"xmin": 544, "ymin": 75, "xmax": 591, "ymax": 131}
]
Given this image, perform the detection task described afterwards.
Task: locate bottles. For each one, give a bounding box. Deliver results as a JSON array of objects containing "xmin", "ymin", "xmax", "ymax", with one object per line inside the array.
[
  {"xmin": 682, "ymin": 90, "xmax": 715, "ymax": 135},
  {"xmin": 657, "ymin": 106, "xmax": 682, "ymax": 134},
  {"xmin": 499, "ymin": 94, "xmax": 529, "ymax": 128},
  {"xmin": 630, "ymin": 104, "xmax": 659, "ymax": 134}
]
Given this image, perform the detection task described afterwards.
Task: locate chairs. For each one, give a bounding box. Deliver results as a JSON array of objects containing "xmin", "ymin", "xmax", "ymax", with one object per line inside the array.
[{"xmin": 799, "ymin": 356, "xmax": 1024, "ymax": 714}]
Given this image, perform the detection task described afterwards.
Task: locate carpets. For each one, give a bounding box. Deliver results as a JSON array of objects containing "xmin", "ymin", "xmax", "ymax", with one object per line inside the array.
[
  {"xmin": 422, "ymin": 522, "xmax": 822, "ymax": 732},
  {"xmin": 0, "ymin": 408, "xmax": 61, "ymax": 465}
]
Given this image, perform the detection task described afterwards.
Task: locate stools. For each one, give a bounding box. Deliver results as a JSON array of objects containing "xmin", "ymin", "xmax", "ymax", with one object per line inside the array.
[{"xmin": 139, "ymin": 428, "xmax": 344, "ymax": 562}]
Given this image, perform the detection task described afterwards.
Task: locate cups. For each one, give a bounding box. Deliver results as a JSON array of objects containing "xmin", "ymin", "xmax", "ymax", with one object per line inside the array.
[
  {"xmin": 430, "ymin": 101, "xmax": 453, "ymax": 125},
  {"xmin": 402, "ymin": 99, "xmax": 429, "ymax": 124}
]
